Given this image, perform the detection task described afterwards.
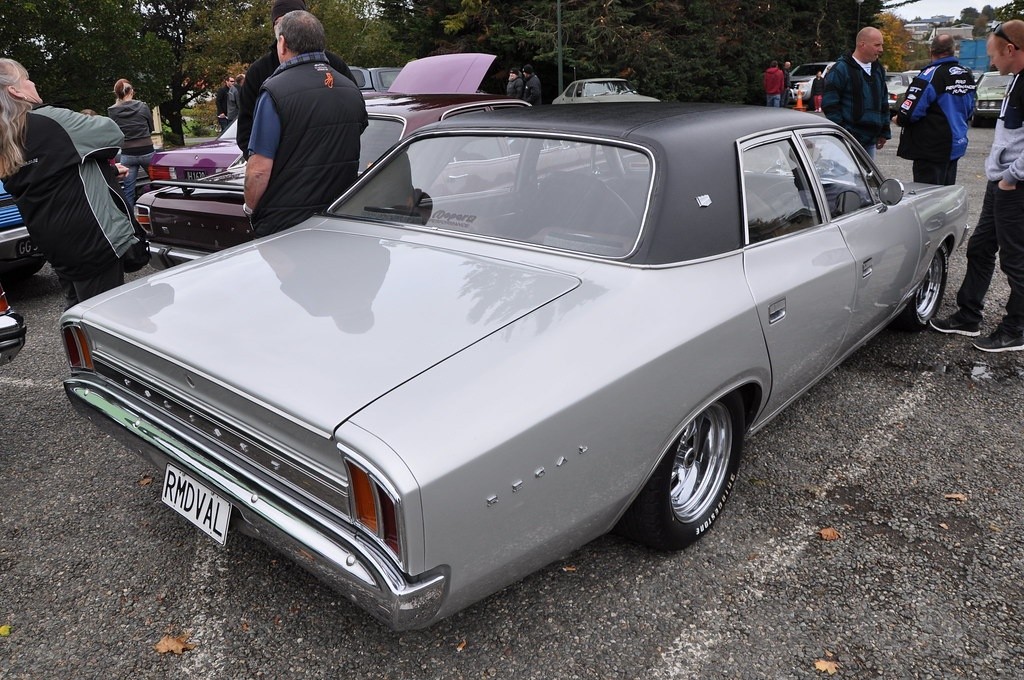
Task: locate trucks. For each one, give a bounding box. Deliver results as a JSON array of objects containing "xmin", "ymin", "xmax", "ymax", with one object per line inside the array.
[{"xmin": 959, "ymin": 40, "xmax": 991, "ymax": 72}]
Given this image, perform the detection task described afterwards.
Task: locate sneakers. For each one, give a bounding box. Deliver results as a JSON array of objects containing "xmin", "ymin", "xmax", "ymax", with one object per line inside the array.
[
  {"xmin": 971, "ymin": 328, "xmax": 1024, "ymax": 352},
  {"xmin": 929, "ymin": 306, "xmax": 983, "ymax": 336}
]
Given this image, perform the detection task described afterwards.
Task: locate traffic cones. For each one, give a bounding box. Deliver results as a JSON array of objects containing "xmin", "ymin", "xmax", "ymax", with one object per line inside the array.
[{"xmin": 793, "ymin": 91, "xmax": 807, "ymax": 112}]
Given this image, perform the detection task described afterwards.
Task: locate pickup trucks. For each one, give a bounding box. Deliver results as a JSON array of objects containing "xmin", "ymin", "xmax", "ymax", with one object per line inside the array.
[{"xmin": 348, "ymin": 66, "xmax": 402, "ymax": 94}]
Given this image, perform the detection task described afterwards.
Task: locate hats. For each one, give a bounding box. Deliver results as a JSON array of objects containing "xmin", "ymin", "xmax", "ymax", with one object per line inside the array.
[
  {"xmin": 509, "ymin": 68, "xmax": 519, "ymax": 75},
  {"xmin": 272, "ymin": 0, "xmax": 306, "ymax": 29},
  {"xmin": 522, "ymin": 64, "xmax": 533, "ymax": 74}
]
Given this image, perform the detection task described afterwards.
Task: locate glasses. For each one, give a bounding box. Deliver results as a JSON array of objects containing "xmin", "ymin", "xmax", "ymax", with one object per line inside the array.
[
  {"xmin": 993, "ymin": 23, "xmax": 1020, "ymax": 50},
  {"xmin": 230, "ymin": 80, "xmax": 235, "ymax": 82}
]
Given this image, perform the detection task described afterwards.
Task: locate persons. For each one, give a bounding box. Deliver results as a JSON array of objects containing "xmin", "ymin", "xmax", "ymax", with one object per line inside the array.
[
  {"xmin": 242, "ymin": 10, "xmax": 370, "ymax": 240},
  {"xmin": 929, "ymin": 20, "xmax": 1024, "ymax": 352},
  {"xmin": 507, "ymin": 68, "xmax": 524, "ymax": 99},
  {"xmin": 789, "ymin": 139, "xmax": 879, "ymax": 213},
  {"xmin": 892, "ymin": 34, "xmax": 976, "ymax": 186},
  {"xmin": 227, "ymin": 74, "xmax": 245, "ymax": 125},
  {"xmin": 523, "ymin": 64, "xmax": 541, "ymax": 106},
  {"xmin": 811, "ymin": 26, "xmax": 892, "ymax": 162},
  {"xmin": 236, "ymin": 0, "xmax": 365, "ymax": 162},
  {"xmin": 0, "ymin": 57, "xmax": 155, "ymax": 312},
  {"xmin": 764, "ymin": 60, "xmax": 784, "ymax": 107},
  {"xmin": 215, "ymin": 76, "xmax": 234, "ymax": 131},
  {"xmin": 780, "ymin": 62, "xmax": 791, "ymax": 107}
]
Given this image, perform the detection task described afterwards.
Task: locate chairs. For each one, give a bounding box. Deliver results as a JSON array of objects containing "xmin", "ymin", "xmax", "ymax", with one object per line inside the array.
[{"xmin": 512, "ymin": 169, "xmax": 639, "ymax": 244}]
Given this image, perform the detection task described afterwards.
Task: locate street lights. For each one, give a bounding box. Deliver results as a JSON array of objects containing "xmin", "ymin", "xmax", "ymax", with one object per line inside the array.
[{"xmin": 856, "ymin": 0, "xmax": 864, "ymax": 34}]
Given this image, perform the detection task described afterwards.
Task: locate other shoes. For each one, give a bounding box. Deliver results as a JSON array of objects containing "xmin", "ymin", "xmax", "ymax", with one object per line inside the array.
[{"xmin": 814, "ymin": 108, "xmax": 821, "ymax": 112}]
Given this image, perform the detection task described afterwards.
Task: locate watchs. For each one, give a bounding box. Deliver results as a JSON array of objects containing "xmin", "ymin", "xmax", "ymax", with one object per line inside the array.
[{"xmin": 242, "ymin": 203, "xmax": 255, "ymax": 215}]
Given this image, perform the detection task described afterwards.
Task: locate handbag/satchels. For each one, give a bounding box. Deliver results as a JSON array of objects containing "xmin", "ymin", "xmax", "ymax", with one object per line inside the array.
[{"xmin": 124, "ymin": 240, "xmax": 150, "ymax": 273}]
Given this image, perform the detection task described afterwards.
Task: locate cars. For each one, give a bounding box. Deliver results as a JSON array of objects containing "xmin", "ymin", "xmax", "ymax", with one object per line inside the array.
[
  {"xmin": 789, "ymin": 62, "xmax": 837, "ymax": 103},
  {"xmin": 969, "ymin": 72, "xmax": 1014, "ymax": 127},
  {"xmin": 552, "ymin": 79, "xmax": 661, "ymax": 104},
  {"xmin": 133, "ymin": 94, "xmax": 647, "ymax": 269},
  {"xmin": 884, "ymin": 70, "xmax": 921, "ymax": 118},
  {"xmin": 58, "ymin": 94, "xmax": 971, "ymax": 635},
  {"xmin": 0, "ymin": 179, "xmax": 46, "ymax": 276},
  {"xmin": 147, "ymin": 54, "xmax": 500, "ymax": 190}
]
